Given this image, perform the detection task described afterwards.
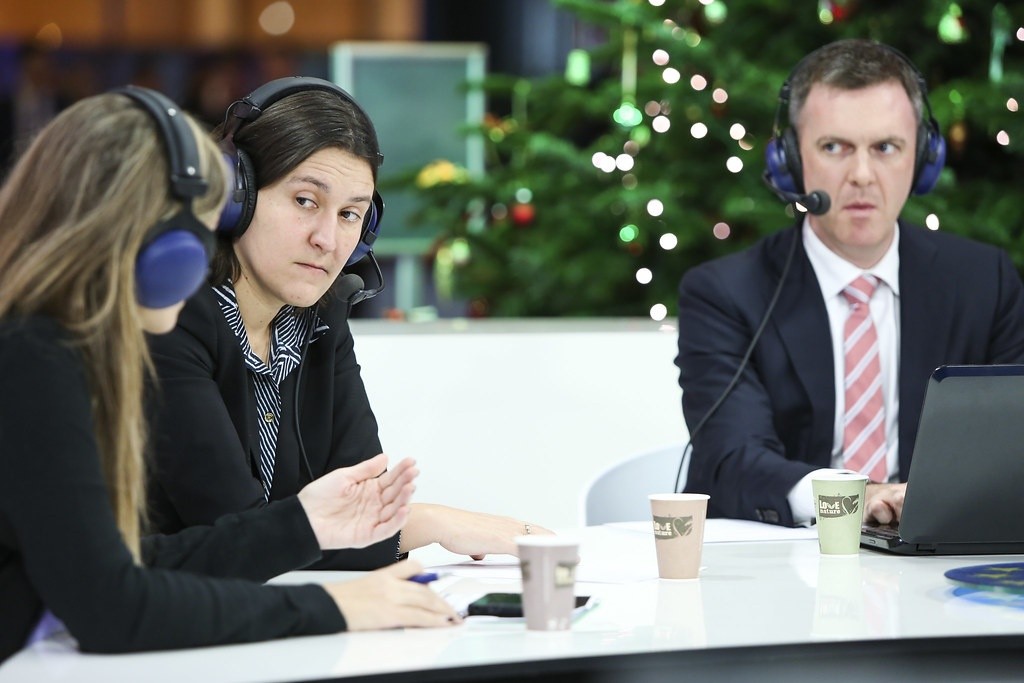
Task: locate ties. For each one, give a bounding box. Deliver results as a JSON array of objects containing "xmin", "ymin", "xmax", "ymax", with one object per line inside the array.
[{"xmin": 842, "ymin": 273, "xmax": 888, "ymax": 484}]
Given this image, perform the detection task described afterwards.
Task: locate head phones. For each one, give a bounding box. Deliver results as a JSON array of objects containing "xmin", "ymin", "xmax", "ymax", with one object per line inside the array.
[
  {"xmin": 213, "ymin": 75, "xmax": 385, "ymax": 265},
  {"xmin": 108, "ymin": 81, "xmax": 218, "ymax": 310},
  {"xmin": 762, "ymin": 46, "xmax": 946, "ymax": 203}
]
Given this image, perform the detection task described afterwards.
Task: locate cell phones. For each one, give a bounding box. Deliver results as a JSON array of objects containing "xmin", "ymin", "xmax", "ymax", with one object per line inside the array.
[{"xmin": 467, "ymin": 591, "xmax": 589, "ymax": 616}]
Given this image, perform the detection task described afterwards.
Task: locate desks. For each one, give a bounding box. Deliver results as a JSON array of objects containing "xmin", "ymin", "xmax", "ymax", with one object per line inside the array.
[{"xmin": 0, "ymin": 521, "xmax": 1024, "ymax": 683}]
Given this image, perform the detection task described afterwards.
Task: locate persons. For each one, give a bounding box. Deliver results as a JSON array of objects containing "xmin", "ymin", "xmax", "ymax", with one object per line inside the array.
[
  {"xmin": 137, "ymin": 77, "xmax": 575, "ymax": 574},
  {"xmin": 679, "ymin": 41, "xmax": 1023, "ymax": 536},
  {"xmin": 0, "ymin": 86, "xmax": 465, "ymax": 658}
]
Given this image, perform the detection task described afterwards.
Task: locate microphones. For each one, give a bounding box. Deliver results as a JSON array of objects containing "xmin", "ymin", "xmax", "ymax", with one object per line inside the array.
[
  {"xmin": 335, "ymin": 252, "xmax": 385, "ymax": 305},
  {"xmin": 764, "ymin": 178, "xmax": 831, "ymax": 214}
]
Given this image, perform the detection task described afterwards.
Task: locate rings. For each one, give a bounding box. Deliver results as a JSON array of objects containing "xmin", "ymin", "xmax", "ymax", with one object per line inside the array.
[{"xmin": 525, "ymin": 525, "xmax": 531, "ymax": 533}]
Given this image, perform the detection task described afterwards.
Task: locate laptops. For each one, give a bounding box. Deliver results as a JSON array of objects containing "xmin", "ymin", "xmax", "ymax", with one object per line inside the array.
[{"xmin": 859, "ymin": 363, "xmax": 1024, "ymax": 556}]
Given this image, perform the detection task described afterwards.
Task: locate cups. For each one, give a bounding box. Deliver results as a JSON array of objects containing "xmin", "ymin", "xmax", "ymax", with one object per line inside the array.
[
  {"xmin": 516, "ymin": 535, "xmax": 581, "ymax": 631},
  {"xmin": 649, "ymin": 493, "xmax": 710, "ymax": 579},
  {"xmin": 812, "ymin": 474, "xmax": 869, "ymax": 555}
]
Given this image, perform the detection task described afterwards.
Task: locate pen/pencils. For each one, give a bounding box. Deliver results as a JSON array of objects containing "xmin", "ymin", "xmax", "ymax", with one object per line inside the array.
[{"xmin": 407, "ymin": 567, "xmax": 461, "ymax": 585}]
